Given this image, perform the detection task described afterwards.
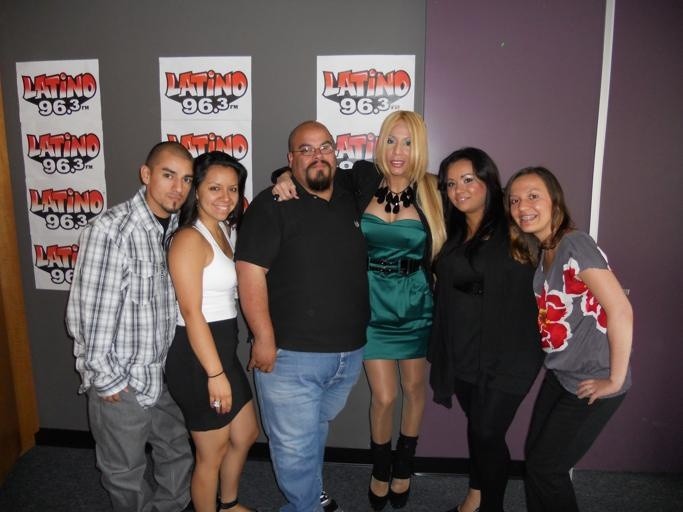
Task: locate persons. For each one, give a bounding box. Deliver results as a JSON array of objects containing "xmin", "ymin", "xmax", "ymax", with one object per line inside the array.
[
  {"xmin": 65, "ymin": 140, "xmax": 194, "ymax": 512},
  {"xmin": 233, "ymin": 121, "xmax": 367, "ymax": 511},
  {"xmin": 503, "ymin": 166, "xmax": 633, "ymax": 512},
  {"xmin": 425, "ymin": 148, "xmax": 541, "ymax": 511},
  {"xmin": 164, "ymin": 151, "xmax": 261, "ymax": 511},
  {"xmin": 270, "ymin": 112, "xmax": 447, "ymax": 510}
]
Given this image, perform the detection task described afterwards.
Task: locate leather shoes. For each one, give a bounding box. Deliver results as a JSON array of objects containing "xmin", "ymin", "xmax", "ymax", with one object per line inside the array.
[
  {"xmin": 369, "ymin": 476, "xmax": 389, "ymax": 512},
  {"xmin": 390, "ymin": 478, "xmax": 411, "ymax": 509}
]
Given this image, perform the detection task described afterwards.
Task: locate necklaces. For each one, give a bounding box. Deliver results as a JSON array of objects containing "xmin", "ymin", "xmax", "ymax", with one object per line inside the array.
[{"xmin": 374, "ymin": 177, "xmax": 418, "ymax": 215}]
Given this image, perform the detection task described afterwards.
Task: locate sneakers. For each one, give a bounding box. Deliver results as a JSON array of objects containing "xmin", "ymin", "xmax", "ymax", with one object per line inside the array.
[{"xmin": 321, "ymin": 493, "xmax": 342, "ymax": 512}]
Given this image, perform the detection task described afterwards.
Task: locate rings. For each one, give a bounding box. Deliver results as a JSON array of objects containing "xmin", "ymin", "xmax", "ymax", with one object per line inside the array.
[
  {"xmin": 273, "ymin": 193, "xmax": 280, "ymax": 201},
  {"xmin": 212, "ymin": 400, "xmax": 222, "ymax": 409},
  {"xmin": 590, "ymin": 389, "xmax": 593, "ymax": 393}
]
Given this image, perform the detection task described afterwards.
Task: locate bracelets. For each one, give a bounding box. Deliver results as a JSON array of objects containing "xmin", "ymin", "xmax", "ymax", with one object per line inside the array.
[{"xmin": 208, "ymin": 370, "xmax": 224, "ymax": 379}]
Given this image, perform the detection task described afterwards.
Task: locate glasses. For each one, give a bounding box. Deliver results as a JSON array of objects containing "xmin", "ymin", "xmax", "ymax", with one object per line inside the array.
[{"xmin": 293, "ymin": 144, "xmax": 335, "ymax": 156}]
